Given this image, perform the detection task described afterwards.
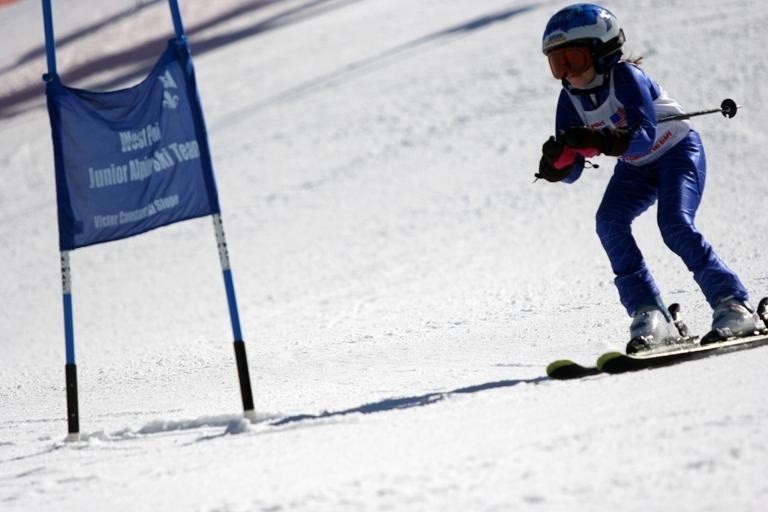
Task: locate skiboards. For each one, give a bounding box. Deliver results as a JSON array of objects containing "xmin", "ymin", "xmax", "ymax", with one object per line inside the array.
[{"xmin": 546, "ymin": 296, "xmax": 767, "ymax": 379}]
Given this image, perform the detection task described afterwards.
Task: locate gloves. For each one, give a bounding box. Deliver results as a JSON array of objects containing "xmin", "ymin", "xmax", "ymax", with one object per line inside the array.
[{"xmin": 535, "ymin": 126, "xmax": 632, "ymax": 183}]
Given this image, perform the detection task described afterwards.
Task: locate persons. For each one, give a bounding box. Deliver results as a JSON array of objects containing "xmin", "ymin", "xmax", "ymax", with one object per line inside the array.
[{"xmin": 540, "ymin": 4, "xmax": 767, "ymax": 351}]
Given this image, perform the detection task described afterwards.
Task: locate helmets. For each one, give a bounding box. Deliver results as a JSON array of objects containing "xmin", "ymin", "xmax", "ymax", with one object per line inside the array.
[{"xmin": 542, "ymin": 3, "xmax": 625, "ymax": 74}]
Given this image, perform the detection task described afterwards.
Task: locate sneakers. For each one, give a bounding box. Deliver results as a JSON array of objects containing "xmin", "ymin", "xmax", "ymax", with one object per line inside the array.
[
  {"xmin": 626, "ymin": 295, "xmax": 683, "ymax": 353},
  {"xmin": 699, "ymin": 294, "xmax": 766, "ymax": 346}
]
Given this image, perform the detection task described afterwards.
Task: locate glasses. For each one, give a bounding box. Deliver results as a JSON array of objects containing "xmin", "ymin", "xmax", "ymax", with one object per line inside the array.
[{"xmin": 546, "ymin": 28, "xmax": 625, "ymax": 79}]
